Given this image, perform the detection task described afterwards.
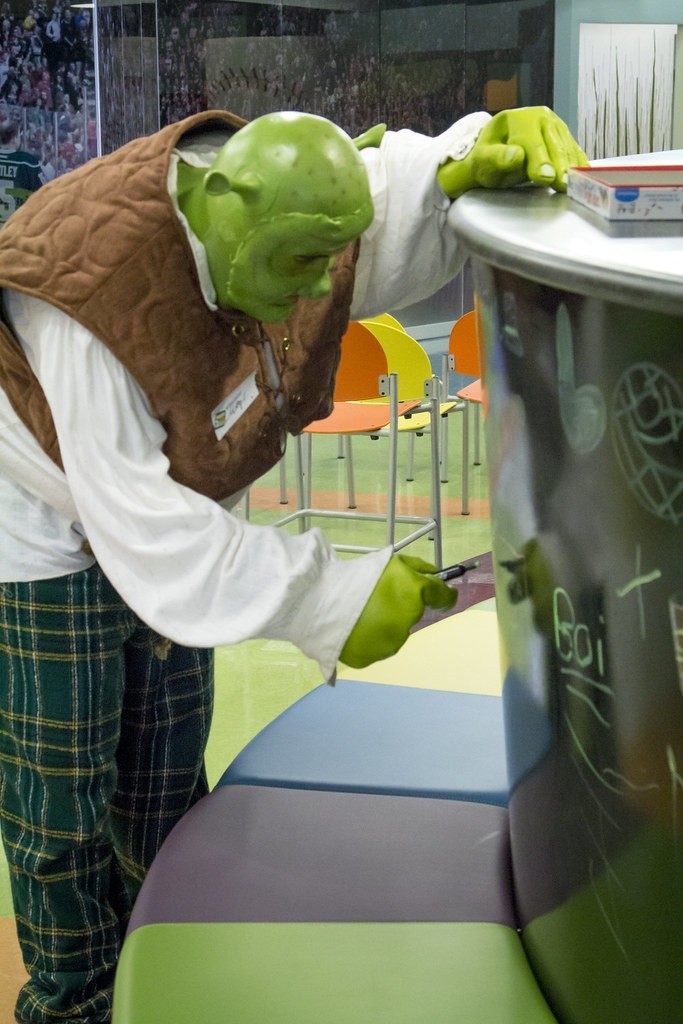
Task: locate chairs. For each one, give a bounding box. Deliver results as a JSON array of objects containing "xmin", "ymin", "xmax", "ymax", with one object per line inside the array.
[{"xmin": 241, "ymin": 310, "xmax": 482, "ymax": 569}]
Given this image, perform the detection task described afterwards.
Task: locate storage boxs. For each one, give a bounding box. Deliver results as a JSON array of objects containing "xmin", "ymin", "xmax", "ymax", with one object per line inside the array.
[{"xmin": 567, "ymin": 169, "xmax": 683, "ymax": 221}]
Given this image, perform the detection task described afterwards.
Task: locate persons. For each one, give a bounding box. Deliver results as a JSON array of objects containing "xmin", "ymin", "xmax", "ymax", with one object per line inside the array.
[
  {"xmin": 0, "ymin": 104, "xmax": 592, "ymax": 1024},
  {"xmin": 0, "ymin": 117, "xmax": 50, "ymax": 227},
  {"xmin": 0, "ymin": 0, "xmax": 97, "ymax": 178}
]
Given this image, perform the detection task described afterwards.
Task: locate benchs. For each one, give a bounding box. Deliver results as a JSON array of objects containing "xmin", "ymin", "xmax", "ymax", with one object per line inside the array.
[{"xmin": 113, "ymin": 552, "xmax": 558, "ymax": 1024}]
